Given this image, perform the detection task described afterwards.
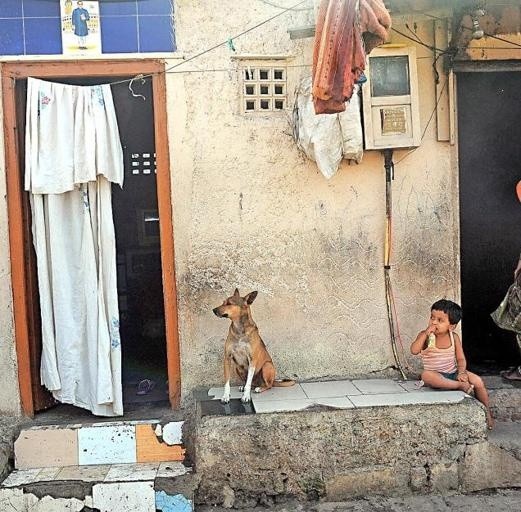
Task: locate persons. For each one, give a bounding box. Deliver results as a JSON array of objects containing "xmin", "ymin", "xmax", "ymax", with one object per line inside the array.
[
  {"xmin": 410, "ymin": 299, "xmax": 493, "ymax": 429},
  {"xmin": 490, "ymin": 252, "xmax": 521, "ymax": 380},
  {"xmin": 72, "ymin": 1, "xmax": 90, "ymax": 49}
]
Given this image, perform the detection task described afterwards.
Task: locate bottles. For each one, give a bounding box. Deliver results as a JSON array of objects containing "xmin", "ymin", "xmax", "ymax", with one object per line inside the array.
[{"xmin": 427, "ymin": 332, "xmax": 436, "ymax": 350}]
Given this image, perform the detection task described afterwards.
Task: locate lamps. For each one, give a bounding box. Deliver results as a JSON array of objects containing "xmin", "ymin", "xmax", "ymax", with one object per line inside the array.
[{"xmin": 472, "ymin": 15, "xmax": 484, "ymax": 39}]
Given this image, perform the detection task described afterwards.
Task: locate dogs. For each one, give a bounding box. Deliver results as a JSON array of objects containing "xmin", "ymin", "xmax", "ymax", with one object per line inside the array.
[{"xmin": 211, "ymin": 287, "xmax": 296, "ymax": 405}]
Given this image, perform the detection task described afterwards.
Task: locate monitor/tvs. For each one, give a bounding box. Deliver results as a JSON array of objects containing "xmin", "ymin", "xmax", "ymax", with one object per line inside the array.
[{"xmin": 136, "ymin": 206, "xmax": 159, "ymax": 245}]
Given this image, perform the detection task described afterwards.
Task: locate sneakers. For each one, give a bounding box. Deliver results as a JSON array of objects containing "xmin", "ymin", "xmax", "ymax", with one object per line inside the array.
[{"xmin": 501, "ymin": 366, "xmax": 521, "ymax": 380}]
